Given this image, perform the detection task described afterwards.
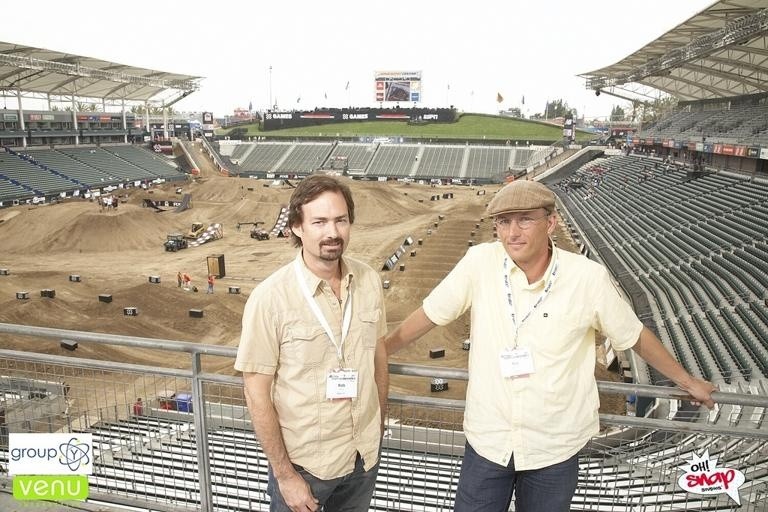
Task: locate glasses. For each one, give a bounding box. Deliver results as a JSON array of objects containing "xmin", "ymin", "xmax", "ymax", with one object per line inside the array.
[{"xmin": 494, "ymin": 214, "xmax": 548, "ymax": 231}]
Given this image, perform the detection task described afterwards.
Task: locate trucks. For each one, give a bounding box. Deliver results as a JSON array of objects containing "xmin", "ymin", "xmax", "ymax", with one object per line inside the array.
[{"xmin": 97, "ymin": 193, "xmax": 117, "ymax": 207}]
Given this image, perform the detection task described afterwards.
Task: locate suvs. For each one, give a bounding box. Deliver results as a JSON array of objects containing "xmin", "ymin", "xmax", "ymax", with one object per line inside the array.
[{"xmin": 249, "ymin": 226, "xmax": 270, "ymax": 240}]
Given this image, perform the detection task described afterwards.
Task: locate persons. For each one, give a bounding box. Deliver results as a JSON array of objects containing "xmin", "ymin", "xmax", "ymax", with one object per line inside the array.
[
  {"xmin": 381, "ymin": 178, "xmax": 720, "ymax": 512},
  {"xmin": 556, "ymin": 135, "xmax": 706, "ymax": 200},
  {"xmin": 182, "ymin": 272, "xmax": 191, "ymax": 290},
  {"xmin": 233, "ymin": 175, "xmax": 389, "ymax": 510},
  {"xmin": 176, "ymin": 271, "xmax": 183, "ymax": 288},
  {"xmin": 206, "ymin": 274, "xmax": 215, "ymax": 294}
]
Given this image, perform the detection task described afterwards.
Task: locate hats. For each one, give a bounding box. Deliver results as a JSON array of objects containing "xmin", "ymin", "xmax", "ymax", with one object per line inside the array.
[{"xmin": 486, "ymin": 180, "xmax": 556, "ymax": 217}]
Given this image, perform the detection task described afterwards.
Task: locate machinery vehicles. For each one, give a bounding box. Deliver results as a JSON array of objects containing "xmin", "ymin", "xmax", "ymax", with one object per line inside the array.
[
  {"xmin": 163, "ymin": 232, "xmax": 187, "ymax": 253},
  {"xmin": 184, "ymin": 222, "xmax": 205, "ymax": 241}
]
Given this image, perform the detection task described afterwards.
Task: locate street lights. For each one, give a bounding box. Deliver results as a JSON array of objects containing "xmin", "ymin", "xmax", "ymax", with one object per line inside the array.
[{"xmin": 267, "ymin": 64, "xmax": 273, "ymax": 111}]
